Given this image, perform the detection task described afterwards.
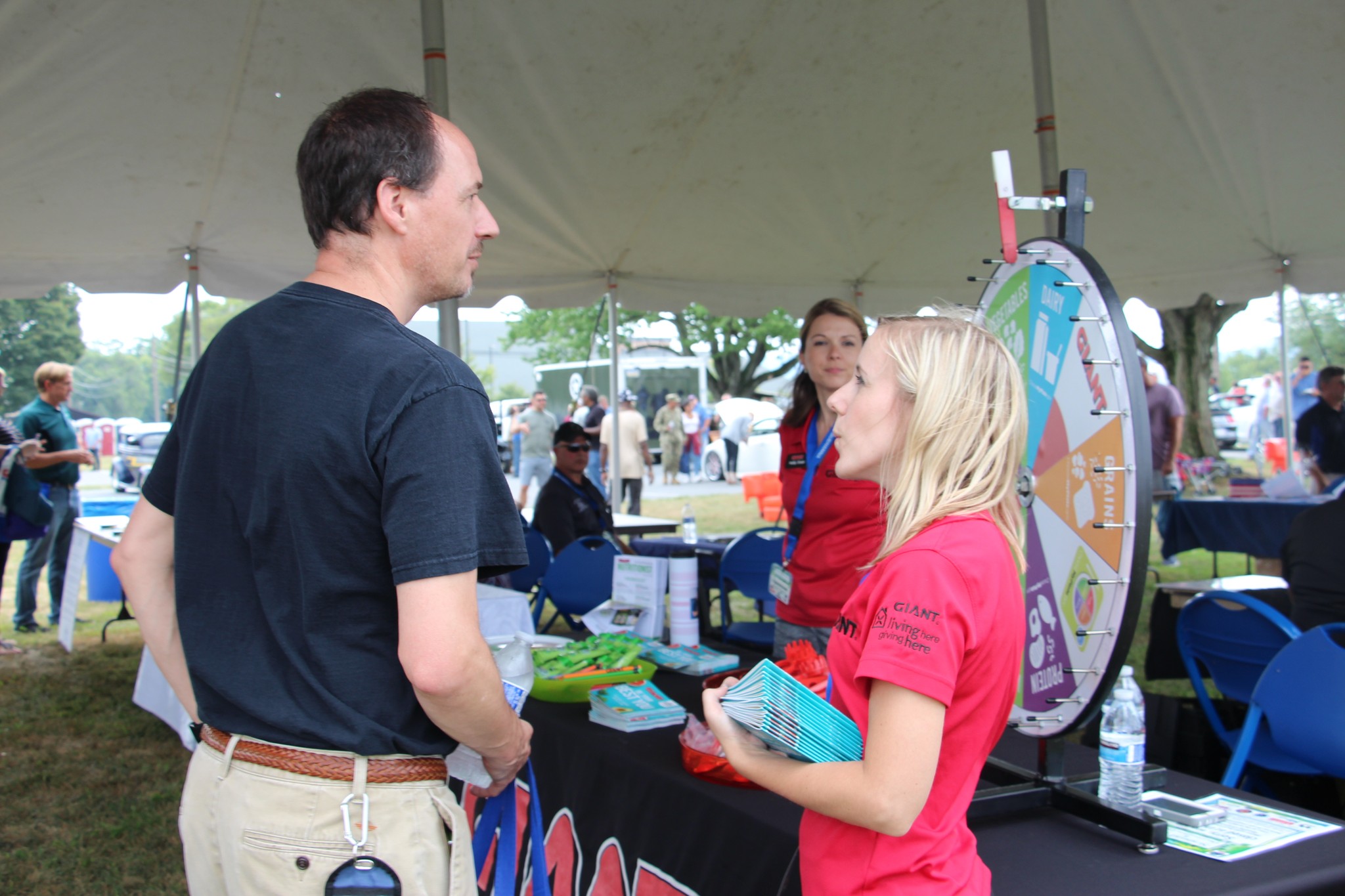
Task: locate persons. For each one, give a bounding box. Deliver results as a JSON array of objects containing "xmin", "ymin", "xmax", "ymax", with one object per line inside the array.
[
  {"xmin": 530, "ymin": 421, "xmax": 637, "ymax": 559},
  {"xmin": 509, "ymin": 390, "xmax": 560, "ymax": 508},
  {"xmin": 1139, "ymin": 356, "xmax": 1185, "ymax": 567},
  {"xmin": 1291, "ymin": 357, "xmax": 1345, "ymax": 495},
  {"xmin": 701, "ymin": 314, "xmax": 1028, "ymax": 896},
  {"xmin": 652, "ymin": 393, "xmax": 754, "ymax": 486},
  {"xmin": 1207, "ymin": 377, "xmax": 1244, "ymax": 407},
  {"xmin": 1258, "ymin": 372, "xmax": 1284, "ymax": 439},
  {"xmin": 108, "ymin": 89, "xmax": 535, "ymax": 896},
  {"xmin": 565, "ymin": 385, "xmax": 653, "ymax": 541},
  {"xmin": 0, "ymin": 361, "xmax": 96, "ymax": 633},
  {"xmin": 773, "ymin": 297, "xmax": 891, "ymax": 661}
]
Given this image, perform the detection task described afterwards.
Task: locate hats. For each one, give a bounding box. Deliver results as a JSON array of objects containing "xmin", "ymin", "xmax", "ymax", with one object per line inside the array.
[
  {"xmin": 618, "ymin": 389, "xmax": 638, "ymax": 402},
  {"xmin": 665, "ymin": 393, "xmax": 681, "ymax": 403},
  {"xmin": 554, "ymin": 421, "xmax": 590, "ymax": 442}
]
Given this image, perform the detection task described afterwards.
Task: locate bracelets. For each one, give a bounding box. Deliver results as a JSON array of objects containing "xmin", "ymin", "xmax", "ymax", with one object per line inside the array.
[{"xmin": 599, "ymin": 467, "xmax": 607, "ymax": 473}]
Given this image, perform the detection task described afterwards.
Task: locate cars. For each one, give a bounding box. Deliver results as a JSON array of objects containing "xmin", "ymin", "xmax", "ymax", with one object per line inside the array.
[{"xmin": 699, "ymin": 414, "xmax": 782, "ymax": 483}]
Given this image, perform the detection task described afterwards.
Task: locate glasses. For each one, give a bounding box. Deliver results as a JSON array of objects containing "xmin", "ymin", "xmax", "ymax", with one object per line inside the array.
[
  {"xmin": 557, "ymin": 442, "xmax": 590, "ymax": 453},
  {"xmin": 1300, "ymin": 365, "xmax": 1310, "ymax": 369}
]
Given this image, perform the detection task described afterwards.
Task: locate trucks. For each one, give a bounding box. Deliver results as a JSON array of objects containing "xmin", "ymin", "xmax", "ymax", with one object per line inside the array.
[{"xmin": 533, "ymin": 355, "xmax": 706, "ymax": 463}]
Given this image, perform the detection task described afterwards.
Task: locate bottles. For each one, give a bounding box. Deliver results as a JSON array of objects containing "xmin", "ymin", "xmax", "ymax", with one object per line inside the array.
[
  {"xmin": 681, "ymin": 503, "xmax": 698, "ymax": 544},
  {"xmin": 1102, "ymin": 665, "xmax": 1145, "ymax": 723},
  {"xmin": 1097, "ymin": 689, "xmax": 1145, "ymax": 828},
  {"xmin": 443, "ymin": 630, "xmax": 535, "ymax": 790}
]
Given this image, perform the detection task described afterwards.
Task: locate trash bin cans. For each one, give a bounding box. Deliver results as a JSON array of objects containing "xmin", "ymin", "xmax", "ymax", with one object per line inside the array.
[
  {"xmin": 76, "ymin": 416, "xmax": 145, "ymax": 460},
  {"xmin": 78, "ymin": 499, "xmax": 136, "ymax": 604}
]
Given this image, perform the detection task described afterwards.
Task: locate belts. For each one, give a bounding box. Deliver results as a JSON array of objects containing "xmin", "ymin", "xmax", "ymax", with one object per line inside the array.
[{"xmin": 198, "ymin": 724, "xmax": 450, "ymax": 785}]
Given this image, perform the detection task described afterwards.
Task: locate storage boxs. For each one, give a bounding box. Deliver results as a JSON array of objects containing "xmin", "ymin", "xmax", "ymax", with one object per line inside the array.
[{"xmin": 1229, "ymin": 478, "xmax": 1265, "ymax": 496}]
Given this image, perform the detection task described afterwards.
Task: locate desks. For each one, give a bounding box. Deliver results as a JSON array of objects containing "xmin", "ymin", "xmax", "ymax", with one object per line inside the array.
[
  {"xmin": 72, "ymin": 515, "xmax": 136, "ymax": 644},
  {"xmin": 522, "ymin": 508, "xmax": 680, "ymax": 534},
  {"xmin": 447, "ymin": 638, "xmax": 1345, "ymax": 896},
  {"xmin": 130, "ymin": 583, "xmax": 537, "ymax": 751},
  {"xmin": 1145, "ymin": 475, "xmax": 1345, "ymax": 681}
]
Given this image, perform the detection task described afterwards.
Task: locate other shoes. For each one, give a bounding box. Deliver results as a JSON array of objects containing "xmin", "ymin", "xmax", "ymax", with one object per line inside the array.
[{"xmin": 14, "ymin": 620, "xmax": 51, "ymax": 633}]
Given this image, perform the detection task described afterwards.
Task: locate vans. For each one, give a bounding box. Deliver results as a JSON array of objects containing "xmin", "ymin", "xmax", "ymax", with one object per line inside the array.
[{"xmin": 488, "ymin": 398, "xmax": 535, "ymax": 474}]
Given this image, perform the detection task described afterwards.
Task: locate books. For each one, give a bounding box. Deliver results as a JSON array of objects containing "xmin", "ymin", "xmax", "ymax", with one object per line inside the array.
[
  {"xmin": 609, "ymin": 630, "xmax": 739, "ymax": 676},
  {"xmin": 719, "ymin": 657, "xmax": 864, "ymax": 764},
  {"xmin": 588, "ymin": 679, "xmax": 686, "ymax": 732}
]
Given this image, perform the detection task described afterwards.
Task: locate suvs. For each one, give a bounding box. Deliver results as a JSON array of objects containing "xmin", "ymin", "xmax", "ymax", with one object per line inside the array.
[{"xmin": 110, "ymin": 422, "xmax": 174, "ymax": 493}]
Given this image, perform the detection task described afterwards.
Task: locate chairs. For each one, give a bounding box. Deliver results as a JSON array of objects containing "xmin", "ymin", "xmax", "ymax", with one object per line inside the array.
[
  {"xmin": 511, "ymin": 527, "xmax": 787, "ymax": 647},
  {"xmin": 742, "ymin": 472, "xmax": 786, "ymax": 522},
  {"xmin": 1176, "ymin": 591, "xmax": 1345, "ymax": 788}
]
